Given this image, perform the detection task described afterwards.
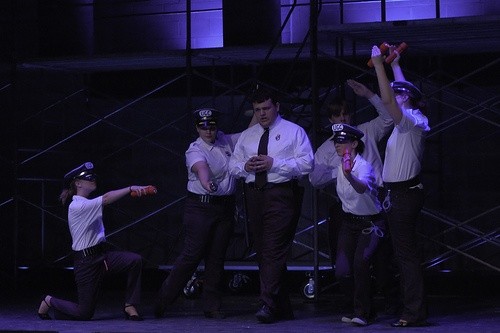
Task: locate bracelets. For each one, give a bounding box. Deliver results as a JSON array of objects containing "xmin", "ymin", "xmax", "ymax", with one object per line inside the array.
[{"xmin": 129, "ymin": 185, "xmax": 132, "ymax": 192}]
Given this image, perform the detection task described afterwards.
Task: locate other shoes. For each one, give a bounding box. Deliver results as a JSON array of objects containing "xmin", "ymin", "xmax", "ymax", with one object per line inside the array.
[
  {"xmin": 352, "ymin": 315, "xmax": 377, "ymax": 325},
  {"xmin": 155, "ymin": 298, "xmax": 165, "ymax": 318},
  {"xmin": 391, "ymin": 318, "xmax": 430, "ymax": 327},
  {"xmin": 342, "ymin": 310, "xmax": 355, "ymax": 322},
  {"xmin": 256, "ymin": 304, "xmax": 274, "ymax": 324},
  {"xmin": 205, "ymin": 311, "xmax": 221, "ymax": 319}
]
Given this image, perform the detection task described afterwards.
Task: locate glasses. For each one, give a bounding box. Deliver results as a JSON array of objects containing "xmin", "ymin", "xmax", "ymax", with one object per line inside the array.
[{"xmin": 200, "ymin": 126, "xmax": 216, "ymax": 131}]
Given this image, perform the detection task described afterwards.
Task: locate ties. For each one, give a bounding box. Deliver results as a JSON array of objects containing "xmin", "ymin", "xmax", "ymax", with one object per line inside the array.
[{"xmin": 255, "ymin": 128, "xmax": 269, "ymax": 192}]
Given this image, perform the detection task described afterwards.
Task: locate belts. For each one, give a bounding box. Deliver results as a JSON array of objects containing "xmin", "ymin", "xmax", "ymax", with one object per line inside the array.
[
  {"xmin": 71, "ymin": 242, "xmax": 108, "ymax": 257},
  {"xmin": 382, "ymin": 175, "xmax": 422, "ymax": 189},
  {"xmin": 337, "ymin": 201, "xmax": 381, "ymax": 224},
  {"xmin": 187, "ymin": 191, "xmax": 234, "ymax": 203}
]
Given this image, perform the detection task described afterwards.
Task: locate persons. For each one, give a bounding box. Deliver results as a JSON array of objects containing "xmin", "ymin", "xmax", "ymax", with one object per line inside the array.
[
  {"xmin": 309, "ymin": 79, "xmax": 395, "ymax": 322},
  {"xmin": 329, "ymin": 123, "xmax": 385, "ymax": 326},
  {"xmin": 37, "ymin": 161, "xmax": 156, "ymax": 321},
  {"xmin": 228, "ymin": 87, "xmax": 314, "ymax": 322},
  {"xmin": 371, "ymin": 45, "xmax": 431, "ymax": 327},
  {"xmin": 150, "ymin": 84, "xmax": 259, "ymax": 319}
]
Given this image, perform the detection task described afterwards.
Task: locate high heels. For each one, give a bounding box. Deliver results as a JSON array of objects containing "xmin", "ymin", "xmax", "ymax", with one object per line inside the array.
[
  {"xmin": 38, "ymin": 294, "xmax": 51, "ymax": 320},
  {"xmin": 121, "ymin": 303, "xmax": 144, "ymax": 321}
]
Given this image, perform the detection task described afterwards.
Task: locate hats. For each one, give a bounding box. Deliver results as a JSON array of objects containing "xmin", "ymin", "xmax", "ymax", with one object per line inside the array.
[
  {"xmin": 390, "ymin": 81, "xmax": 424, "ymax": 104},
  {"xmin": 193, "ymin": 108, "xmax": 222, "ymax": 125},
  {"xmin": 64, "ymin": 161, "xmax": 96, "ymax": 179},
  {"xmin": 329, "ymin": 123, "xmax": 364, "ymax": 142}
]
